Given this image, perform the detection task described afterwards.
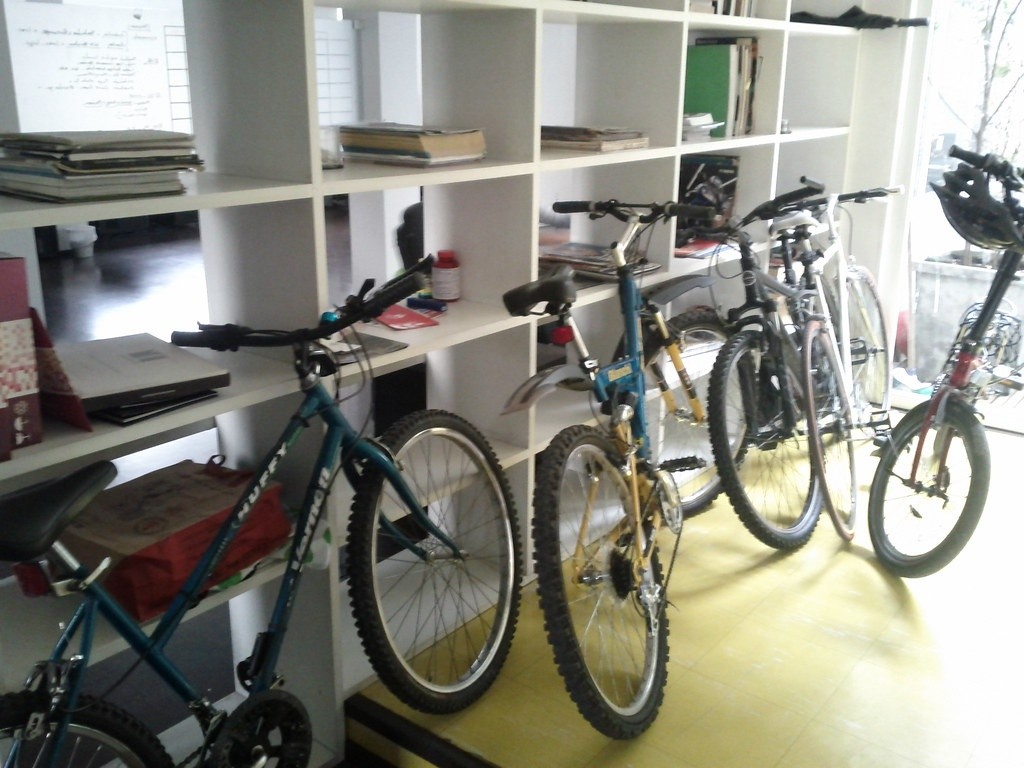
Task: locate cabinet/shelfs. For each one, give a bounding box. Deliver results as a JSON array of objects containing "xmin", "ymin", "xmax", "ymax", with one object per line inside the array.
[{"xmin": 0, "ymin": 1, "xmax": 863, "ymax": 768}]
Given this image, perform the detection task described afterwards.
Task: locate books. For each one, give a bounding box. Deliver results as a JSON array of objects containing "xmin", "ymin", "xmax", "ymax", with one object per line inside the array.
[
  {"xmin": 538, "ymin": 242, "xmax": 661, "ymax": 283},
  {"xmin": 540, "ymin": 126, "xmax": 650, "ymax": 152},
  {"xmin": 683, "ymin": 35, "xmax": 761, "ymax": 139},
  {"xmin": 674, "ymin": 154, "xmax": 740, "ymax": 259},
  {"xmin": 339, "ymin": 122, "xmax": 488, "ymax": 169},
  {"xmin": 0, "ymin": 129, "xmax": 206, "ymax": 205},
  {"xmin": 690, "ymin": 0, "xmax": 757, "ymax": 17}
]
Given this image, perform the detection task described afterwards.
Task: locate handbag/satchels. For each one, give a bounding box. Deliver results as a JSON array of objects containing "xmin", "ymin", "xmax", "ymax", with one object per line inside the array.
[{"xmin": 66, "ymin": 456, "xmax": 293, "ymax": 626}]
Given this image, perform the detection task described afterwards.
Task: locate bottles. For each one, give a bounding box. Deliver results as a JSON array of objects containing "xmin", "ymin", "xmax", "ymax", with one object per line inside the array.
[{"xmin": 431, "ymin": 249, "xmax": 461, "ymax": 303}]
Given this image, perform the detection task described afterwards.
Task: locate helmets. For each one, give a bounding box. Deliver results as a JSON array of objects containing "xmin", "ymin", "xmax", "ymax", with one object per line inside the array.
[{"xmin": 929, "ymin": 164, "xmax": 1023, "ymax": 255}]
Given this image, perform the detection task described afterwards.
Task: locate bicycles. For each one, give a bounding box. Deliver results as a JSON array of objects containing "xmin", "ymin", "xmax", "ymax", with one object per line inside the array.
[
  {"xmin": 775, "ymin": 184, "xmax": 902, "ymax": 543},
  {"xmin": 500, "ymin": 195, "xmax": 759, "ymax": 743},
  {"xmin": 676, "ymin": 172, "xmax": 847, "ymax": 554},
  {"xmin": 866, "ymin": 139, "xmax": 1024, "ymax": 579},
  {"xmin": 0, "ymin": 255, "xmax": 522, "ymax": 768}
]
